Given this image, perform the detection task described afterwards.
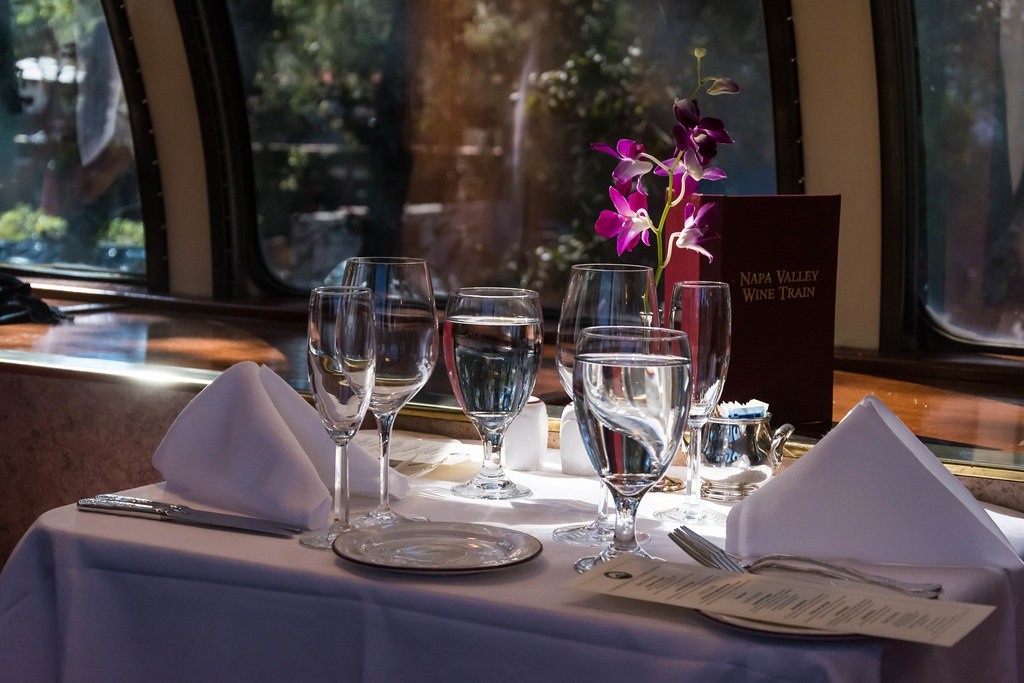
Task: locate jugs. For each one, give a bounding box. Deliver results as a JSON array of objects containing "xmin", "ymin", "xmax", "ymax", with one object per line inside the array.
[{"xmin": 679, "ymin": 412, "xmax": 795, "ymax": 506}]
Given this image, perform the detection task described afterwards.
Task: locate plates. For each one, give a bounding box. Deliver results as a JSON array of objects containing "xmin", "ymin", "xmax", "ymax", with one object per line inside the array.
[
  {"xmin": 691, "ymin": 610, "xmax": 868, "ymax": 642},
  {"xmin": 331, "ymin": 521, "xmax": 543, "ymax": 575}
]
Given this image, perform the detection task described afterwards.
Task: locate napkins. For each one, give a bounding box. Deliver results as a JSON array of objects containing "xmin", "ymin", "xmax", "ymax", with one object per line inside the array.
[
  {"xmin": 724, "ymin": 396, "xmax": 1023, "ymax": 577},
  {"xmin": 152, "ymin": 360, "xmax": 411, "ymax": 532}
]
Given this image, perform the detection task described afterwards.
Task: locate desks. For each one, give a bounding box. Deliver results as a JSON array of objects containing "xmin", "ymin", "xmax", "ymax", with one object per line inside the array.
[{"xmin": 1, "ymin": 430, "xmax": 1024, "ymax": 683}]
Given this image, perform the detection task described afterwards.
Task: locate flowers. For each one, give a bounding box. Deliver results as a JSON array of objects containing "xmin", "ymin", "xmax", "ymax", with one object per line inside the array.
[{"xmin": 589, "ymin": 49, "xmax": 741, "ymax": 316}]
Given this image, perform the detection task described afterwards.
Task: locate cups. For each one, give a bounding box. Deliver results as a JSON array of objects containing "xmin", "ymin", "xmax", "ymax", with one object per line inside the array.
[
  {"xmin": 499, "ymin": 396, "xmax": 547, "ymax": 472},
  {"xmin": 559, "ymin": 401, "xmax": 598, "ymax": 477}
]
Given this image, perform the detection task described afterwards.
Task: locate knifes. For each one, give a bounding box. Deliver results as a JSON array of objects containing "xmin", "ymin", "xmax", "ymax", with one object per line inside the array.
[{"xmin": 76, "ymin": 493, "xmax": 311, "ymax": 538}]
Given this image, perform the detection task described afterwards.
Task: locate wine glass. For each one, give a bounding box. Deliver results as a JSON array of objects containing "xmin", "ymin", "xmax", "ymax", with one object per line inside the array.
[
  {"xmin": 551, "ymin": 263, "xmax": 662, "ymax": 549},
  {"xmin": 340, "ymin": 257, "xmax": 441, "ymax": 527},
  {"xmin": 571, "ymin": 326, "xmax": 693, "ymax": 575},
  {"xmin": 297, "ymin": 285, "xmax": 379, "ymax": 550},
  {"xmin": 443, "ymin": 285, "xmax": 544, "ymax": 501},
  {"xmin": 653, "ymin": 281, "xmax": 732, "ymax": 527}
]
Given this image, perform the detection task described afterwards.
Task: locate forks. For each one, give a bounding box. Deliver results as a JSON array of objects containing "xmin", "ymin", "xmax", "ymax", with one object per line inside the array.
[{"xmin": 668, "ymin": 525, "xmax": 941, "ymax": 601}]
